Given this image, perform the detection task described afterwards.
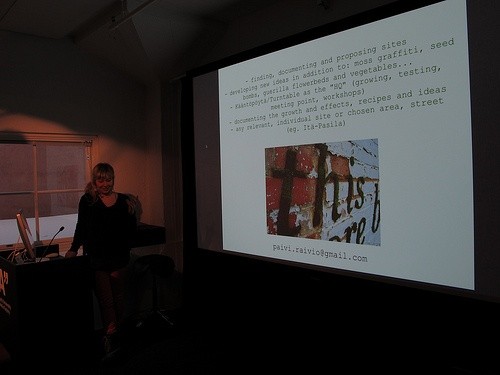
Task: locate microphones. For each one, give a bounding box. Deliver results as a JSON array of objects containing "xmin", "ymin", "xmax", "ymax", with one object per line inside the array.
[{"xmin": 38, "ymin": 225, "xmax": 65, "ymax": 262}]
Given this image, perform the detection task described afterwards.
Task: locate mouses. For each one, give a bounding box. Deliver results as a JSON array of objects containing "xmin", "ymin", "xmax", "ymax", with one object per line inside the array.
[{"xmin": 45, "ymin": 252, "xmax": 58, "ymax": 258}]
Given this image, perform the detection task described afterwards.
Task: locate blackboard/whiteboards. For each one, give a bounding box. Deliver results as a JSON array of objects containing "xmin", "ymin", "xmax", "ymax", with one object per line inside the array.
[{"xmin": 189, "ymin": 0, "xmax": 500, "ymax": 306}]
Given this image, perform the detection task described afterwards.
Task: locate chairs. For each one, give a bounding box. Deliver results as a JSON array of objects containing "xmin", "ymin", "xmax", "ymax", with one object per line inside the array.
[{"xmin": 122, "ymin": 253, "xmax": 174, "ymax": 336}]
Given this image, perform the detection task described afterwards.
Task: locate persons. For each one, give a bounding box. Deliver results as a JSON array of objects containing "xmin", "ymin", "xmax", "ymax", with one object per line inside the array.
[{"xmin": 66, "ymin": 163, "xmax": 138, "ymax": 336}]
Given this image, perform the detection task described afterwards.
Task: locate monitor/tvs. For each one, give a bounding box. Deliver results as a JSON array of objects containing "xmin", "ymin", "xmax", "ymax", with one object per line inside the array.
[{"xmin": 16, "ymin": 208, "xmax": 37, "ymax": 260}]
